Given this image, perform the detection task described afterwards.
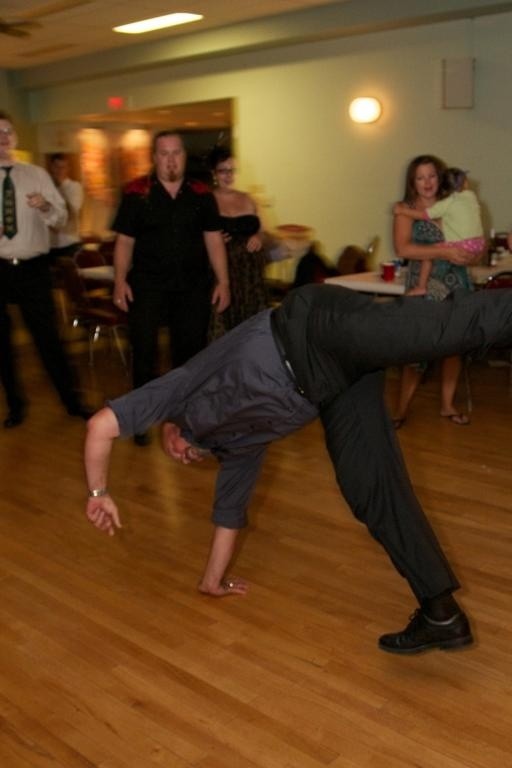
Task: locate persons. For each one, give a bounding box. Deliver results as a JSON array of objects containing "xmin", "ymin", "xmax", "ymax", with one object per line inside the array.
[
  {"xmin": 36, "ymin": 147, "xmax": 88, "ymax": 317},
  {"xmin": 201, "ymin": 144, "xmax": 265, "ymax": 343},
  {"xmin": 391, "ymin": 167, "xmax": 485, "ymax": 298},
  {"xmin": 2, "ymin": 113, "xmax": 98, "ymax": 430},
  {"xmin": 80, "ymin": 279, "xmax": 510, "ymax": 655},
  {"xmin": 384, "ymin": 155, "xmax": 477, "ymax": 429},
  {"xmin": 104, "ymin": 128, "xmax": 235, "ymax": 445}
]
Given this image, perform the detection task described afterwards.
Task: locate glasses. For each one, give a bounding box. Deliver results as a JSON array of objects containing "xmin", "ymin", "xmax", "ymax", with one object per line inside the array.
[
  {"xmin": 216, "ymin": 168, "xmax": 231, "ymax": 174},
  {"xmin": 3, "ymin": 128, "xmax": 13, "ymax": 135}
]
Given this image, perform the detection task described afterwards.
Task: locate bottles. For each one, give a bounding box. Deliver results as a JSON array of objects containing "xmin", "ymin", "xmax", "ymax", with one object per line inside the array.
[{"xmin": 485, "ymin": 228, "xmax": 499, "ymax": 266}]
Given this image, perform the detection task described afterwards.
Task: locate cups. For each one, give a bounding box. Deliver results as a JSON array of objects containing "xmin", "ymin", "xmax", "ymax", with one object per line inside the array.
[
  {"xmin": 391, "ymin": 256, "xmax": 402, "ymax": 277},
  {"xmin": 381, "ymin": 261, "xmax": 395, "ymax": 281}
]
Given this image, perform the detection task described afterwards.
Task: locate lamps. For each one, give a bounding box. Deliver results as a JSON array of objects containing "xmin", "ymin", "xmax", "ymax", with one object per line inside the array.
[{"xmin": 348, "ymin": 96, "xmax": 382, "ymax": 124}]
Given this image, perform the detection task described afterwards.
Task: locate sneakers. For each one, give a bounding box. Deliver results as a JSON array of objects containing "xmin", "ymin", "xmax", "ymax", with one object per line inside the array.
[{"xmin": 134, "ymin": 432, "xmax": 151, "ymax": 447}]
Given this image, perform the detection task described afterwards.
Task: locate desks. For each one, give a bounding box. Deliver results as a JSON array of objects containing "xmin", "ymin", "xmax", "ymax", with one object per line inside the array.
[{"xmin": 324, "ymin": 254, "xmax": 512, "ymax": 412}]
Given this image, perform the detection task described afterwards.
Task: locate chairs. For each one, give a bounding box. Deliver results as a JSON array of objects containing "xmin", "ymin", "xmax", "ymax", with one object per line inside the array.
[{"xmin": 73, "ymin": 249, "xmax": 127, "ymax": 364}]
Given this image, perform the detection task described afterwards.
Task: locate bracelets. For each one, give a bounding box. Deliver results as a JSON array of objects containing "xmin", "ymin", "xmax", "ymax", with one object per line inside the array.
[{"xmin": 88, "ymin": 488, "xmax": 106, "ymax": 497}]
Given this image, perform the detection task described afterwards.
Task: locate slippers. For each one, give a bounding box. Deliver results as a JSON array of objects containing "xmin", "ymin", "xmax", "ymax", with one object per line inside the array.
[
  {"xmin": 439, "ymin": 412, "xmax": 471, "ymax": 426},
  {"xmin": 391, "ymin": 416, "xmax": 407, "ymax": 430}
]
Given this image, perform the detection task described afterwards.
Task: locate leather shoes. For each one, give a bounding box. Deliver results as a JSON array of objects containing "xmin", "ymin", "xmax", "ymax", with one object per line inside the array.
[
  {"xmin": 378, "ymin": 606, "xmax": 474, "ymax": 655},
  {"xmin": 68, "ymin": 405, "xmax": 95, "ymax": 421},
  {"xmin": 3, "ymin": 400, "xmax": 25, "ymax": 428}
]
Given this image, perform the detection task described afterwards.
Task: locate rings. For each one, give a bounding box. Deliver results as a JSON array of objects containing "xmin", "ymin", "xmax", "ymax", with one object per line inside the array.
[
  {"xmin": 118, "ymin": 298, "xmax": 122, "ymax": 303},
  {"xmin": 228, "ymin": 582, "xmax": 235, "ymax": 588}
]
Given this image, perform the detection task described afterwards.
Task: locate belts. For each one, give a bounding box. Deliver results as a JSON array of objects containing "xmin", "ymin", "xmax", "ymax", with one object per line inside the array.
[
  {"xmin": 2, "ymin": 257, "xmax": 34, "ymax": 267},
  {"xmin": 268, "ymin": 307, "xmax": 311, "ymax": 400}
]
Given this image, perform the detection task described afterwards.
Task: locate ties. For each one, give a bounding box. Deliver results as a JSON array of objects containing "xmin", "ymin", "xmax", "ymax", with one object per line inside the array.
[{"xmin": 3, "ymin": 166, "xmax": 18, "ymax": 240}]
